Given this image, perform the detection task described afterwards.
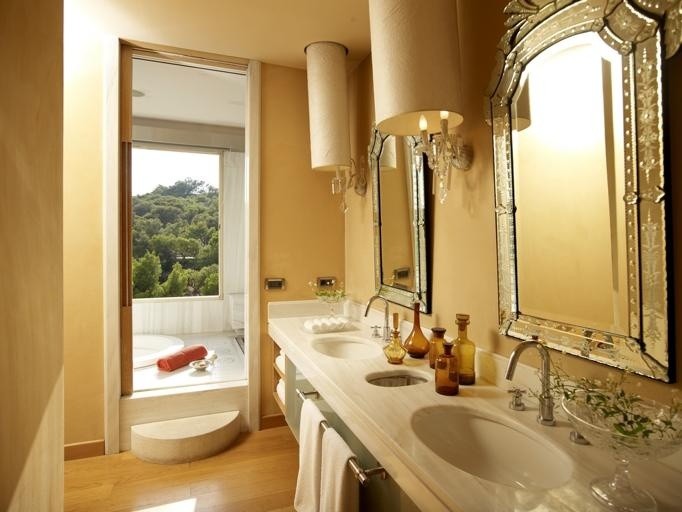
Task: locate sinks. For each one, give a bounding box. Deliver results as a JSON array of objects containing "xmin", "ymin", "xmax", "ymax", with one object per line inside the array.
[
  {"xmin": 410, "ymin": 403, "xmax": 577, "ymax": 490},
  {"xmin": 312, "ymin": 336, "xmax": 383, "ymax": 360}
]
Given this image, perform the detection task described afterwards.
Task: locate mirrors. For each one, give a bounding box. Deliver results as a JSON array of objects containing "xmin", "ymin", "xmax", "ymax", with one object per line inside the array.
[
  {"xmin": 368, "ymin": 127, "xmax": 429, "ymax": 315},
  {"xmin": 483, "ymin": 2, "xmax": 682, "ymax": 384}
]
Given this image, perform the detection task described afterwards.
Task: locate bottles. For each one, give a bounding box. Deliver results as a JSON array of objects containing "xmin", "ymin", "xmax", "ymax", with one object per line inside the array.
[
  {"xmin": 404, "ymin": 303, "xmax": 431, "ymax": 358},
  {"xmin": 435, "ymin": 342, "xmax": 459, "ymax": 395},
  {"xmin": 429, "ymin": 328, "xmax": 447, "ymax": 368},
  {"xmin": 450, "ymin": 313, "xmax": 477, "ymax": 385}
]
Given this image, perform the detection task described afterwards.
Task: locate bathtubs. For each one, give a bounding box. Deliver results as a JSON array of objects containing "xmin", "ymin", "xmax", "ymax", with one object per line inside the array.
[{"xmin": 133, "ymin": 333, "xmax": 185, "ymax": 369}]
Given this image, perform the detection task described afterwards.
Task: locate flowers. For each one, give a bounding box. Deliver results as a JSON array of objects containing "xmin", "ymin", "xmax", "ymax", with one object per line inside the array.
[
  {"xmin": 522, "ymin": 362, "xmax": 679, "ymax": 440},
  {"xmin": 306, "ymin": 279, "xmax": 349, "ymax": 298}
]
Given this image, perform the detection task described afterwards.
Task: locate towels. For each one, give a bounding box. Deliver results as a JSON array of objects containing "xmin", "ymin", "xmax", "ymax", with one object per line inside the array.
[
  {"xmin": 292, "ymin": 398, "xmax": 358, "ymax": 512},
  {"xmin": 303, "ymin": 316, "xmax": 352, "ymax": 333}
]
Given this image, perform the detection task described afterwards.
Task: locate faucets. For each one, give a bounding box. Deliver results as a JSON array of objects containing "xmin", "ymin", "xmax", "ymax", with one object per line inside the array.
[
  {"xmin": 364, "ymin": 294, "xmax": 390, "ymax": 344},
  {"xmin": 506, "ymin": 340, "xmax": 555, "ymax": 424}
]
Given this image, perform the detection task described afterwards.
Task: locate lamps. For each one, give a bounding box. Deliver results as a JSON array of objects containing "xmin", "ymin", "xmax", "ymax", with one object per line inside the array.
[
  {"xmin": 330, "ymin": 158, "xmax": 366, "ymax": 215},
  {"xmin": 413, "ymin": 110, "xmax": 473, "ymax": 204}
]
